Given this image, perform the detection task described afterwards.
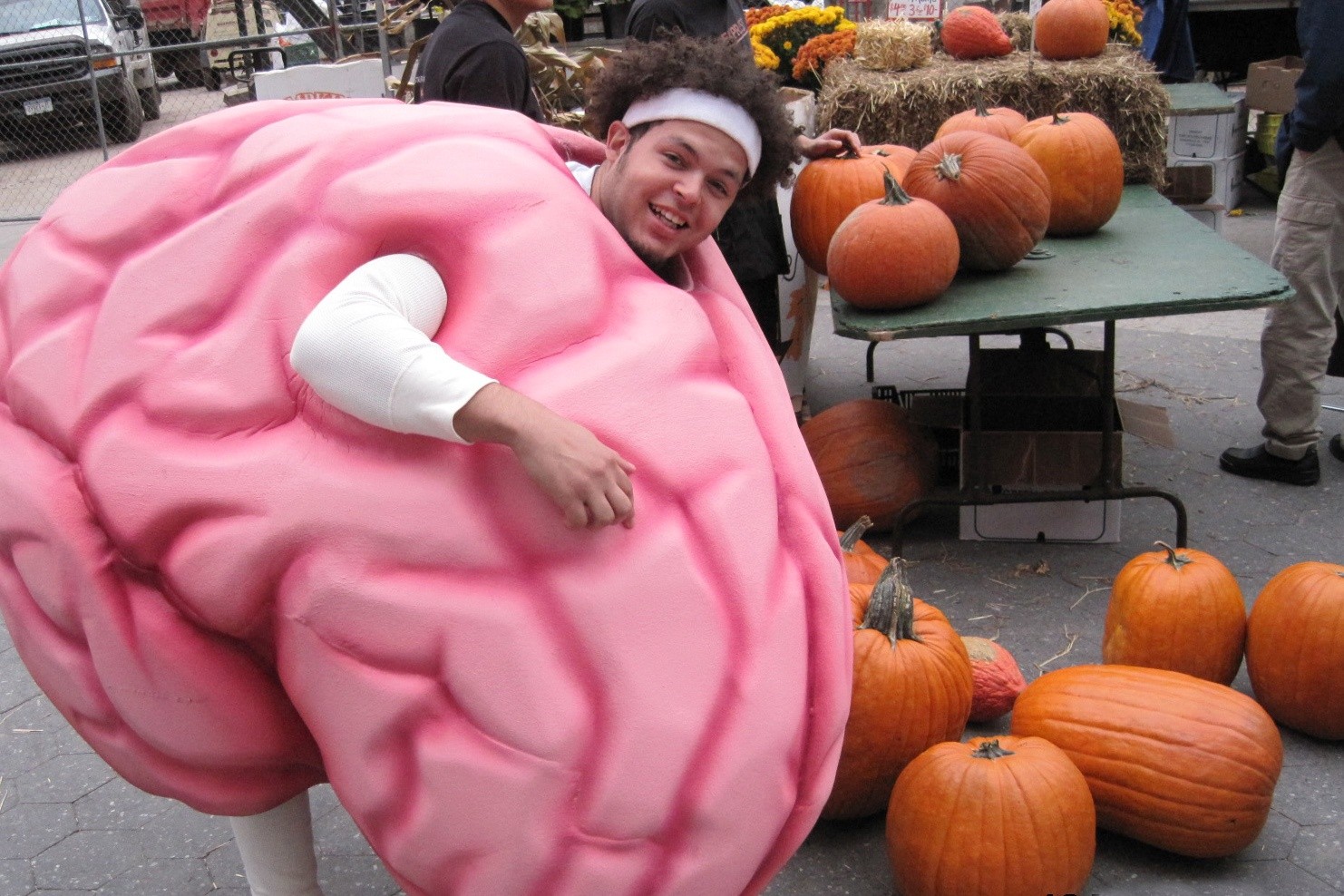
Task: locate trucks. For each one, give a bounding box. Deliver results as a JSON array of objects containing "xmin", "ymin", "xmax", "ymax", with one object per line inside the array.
[{"xmin": 137, "ymin": 0, "xmax": 212, "ymax": 88}]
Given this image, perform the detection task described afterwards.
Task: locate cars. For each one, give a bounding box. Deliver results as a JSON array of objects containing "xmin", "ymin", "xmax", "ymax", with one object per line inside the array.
[{"xmin": 269, "ymin": 0, "xmax": 345, "ymax": 70}]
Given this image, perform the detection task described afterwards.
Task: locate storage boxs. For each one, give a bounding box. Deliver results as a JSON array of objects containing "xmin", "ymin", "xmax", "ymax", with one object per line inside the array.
[
  {"xmin": 1177, "ymin": 194, "xmax": 1225, "ymax": 239},
  {"xmin": 909, "ymin": 348, "xmax": 1173, "ymax": 544},
  {"xmin": 1246, "ymin": 55, "xmax": 1303, "ymax": 113},
  {"xmin": 872, "ymin": 386, "xmax": 965, "ymax": 485},
  {"xmin": 1165, "ymin": 91, "xmax": 1249, "ymax": 160},
  {"xmin": 775, "ymin": 86, "xmax": 817, "ymax": 413},
  {"xmin": 1167, "ymin": 136, "xmax": 1255, "ymax": 211},
  {"xmin": 250, "ymin": 49, "xmax": 420, "ymax": 100}
]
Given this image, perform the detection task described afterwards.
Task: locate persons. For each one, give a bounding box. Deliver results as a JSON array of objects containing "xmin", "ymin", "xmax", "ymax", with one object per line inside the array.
[
  {"xmin": 0, "ymin": 43, "xmax": 854, "ymax": 896},
  {"xmin": 421, "ymin": 0, "xmax": 551, "ymax": 122},
  {"xmin": 626, "ymin": 0, "xmax": 862, "ymax": 352},
  {"xmin": 1219, "ymin": 0, "xmax": 1344, "ymax": 485}
]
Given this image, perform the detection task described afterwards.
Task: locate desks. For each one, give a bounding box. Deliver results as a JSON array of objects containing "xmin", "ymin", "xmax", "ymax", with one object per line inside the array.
[{"xmin": 828, "ymin": 181, "xmax": 1298, "ymax": 561}]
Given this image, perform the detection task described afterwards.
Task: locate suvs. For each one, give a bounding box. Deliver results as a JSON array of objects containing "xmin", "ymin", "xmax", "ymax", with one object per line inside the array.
[{"xmin": 0, "ymin": 0, "xmax": 162, "ymax": 145}]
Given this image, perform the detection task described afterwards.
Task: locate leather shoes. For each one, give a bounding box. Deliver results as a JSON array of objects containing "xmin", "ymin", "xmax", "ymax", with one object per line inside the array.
[
  {"xmin": 1328, "ymin": 432, "xmax": 1344, "ymax": 462},
  {"xmin": 1218, "ymin": 437, "xmax": 1321, "ymax": 488}
]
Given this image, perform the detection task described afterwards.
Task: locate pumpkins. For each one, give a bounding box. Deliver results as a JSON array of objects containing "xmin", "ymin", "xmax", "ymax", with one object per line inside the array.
[
  {"xmin": 1034, "ymin": 0, "xmax": 1108, "ymax": 61},
  {"xmin": 940, "ymin": 5, "xmax": 1022, "ymax": 59},
  {"xmin": 790, "ymin": 89, "xmax": 1123, "ymax": 308},
  {"xmin": 794, "ymin": 398, "xmax": 1344, "ymax": 896}
]
